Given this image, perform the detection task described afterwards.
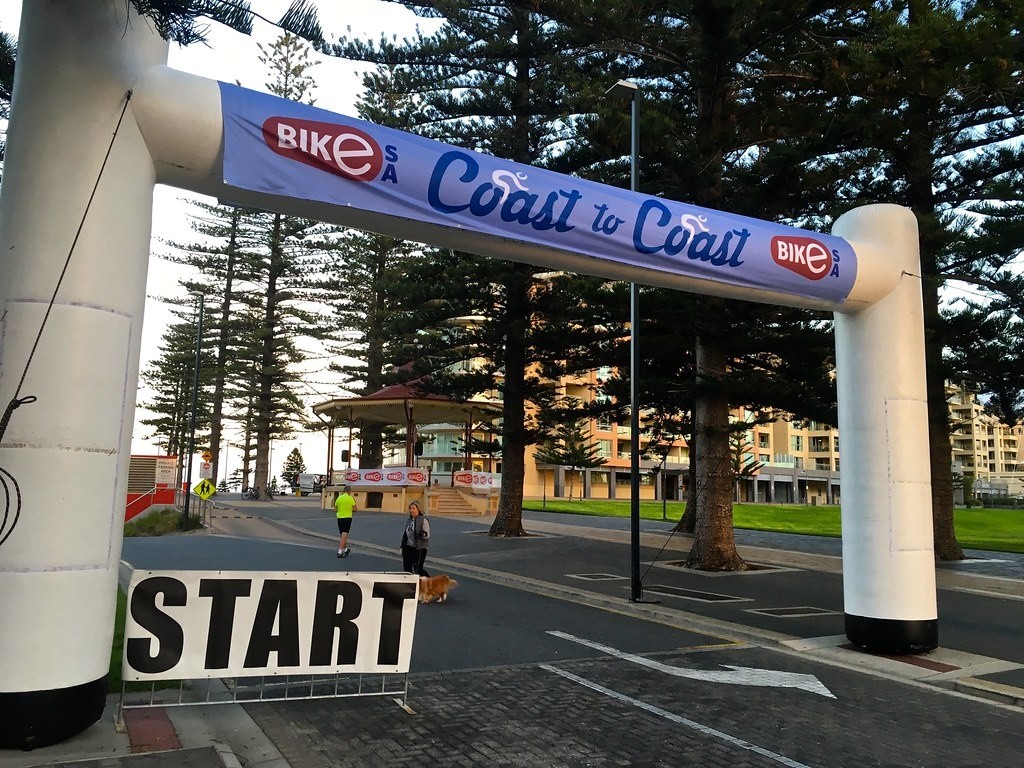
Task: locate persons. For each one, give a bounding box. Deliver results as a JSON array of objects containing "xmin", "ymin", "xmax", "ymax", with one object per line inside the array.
[
  {"xmin": 334, "ymin": 486, "xmax": 357, "ymax": 558},
  {"xmin": 400, "ymin": 500, "xmax": 430, "ymax": 578}
]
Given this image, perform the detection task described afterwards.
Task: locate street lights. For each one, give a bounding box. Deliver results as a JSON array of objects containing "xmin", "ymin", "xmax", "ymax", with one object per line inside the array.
[
  {"xmin": 801, "ymin": 470, "xmax": 808, "ymax": 505},
  {"xmin": 181, "ymin": 290, "xmax": 204, "ymax": 532},
  {"xmin": 605, "ymin": 80, "xmax": 643, "ymax": 602},
  {"xmin": 299, "ymin": 442, "xmax": 302, "ymax": 455}
]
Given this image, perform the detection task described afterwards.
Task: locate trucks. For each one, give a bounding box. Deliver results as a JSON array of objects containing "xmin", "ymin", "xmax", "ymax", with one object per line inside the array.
[{"xmin": 291, "ymin": 473, "xmax": 327, "ymax": 496}]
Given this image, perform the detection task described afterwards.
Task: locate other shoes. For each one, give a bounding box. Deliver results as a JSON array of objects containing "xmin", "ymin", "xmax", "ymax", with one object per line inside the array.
[
  {"xmin": 345, "ymin": 549, "xmax": 350, "ymax": 556},
  {"xmin": 336, "ymin": 553, "xmax": 346, "ymax": 558}
]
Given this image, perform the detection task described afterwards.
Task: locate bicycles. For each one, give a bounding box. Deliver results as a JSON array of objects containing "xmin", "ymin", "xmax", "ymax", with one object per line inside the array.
[{"xmin": 241, "ymin": 486, "xmax": 260, "ymax": 500}]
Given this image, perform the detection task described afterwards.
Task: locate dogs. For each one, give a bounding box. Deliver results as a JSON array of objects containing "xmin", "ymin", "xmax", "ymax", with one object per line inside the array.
[{"xmin": 418, "ymin": 574, "xmax": 459, "ymax": 604}]
